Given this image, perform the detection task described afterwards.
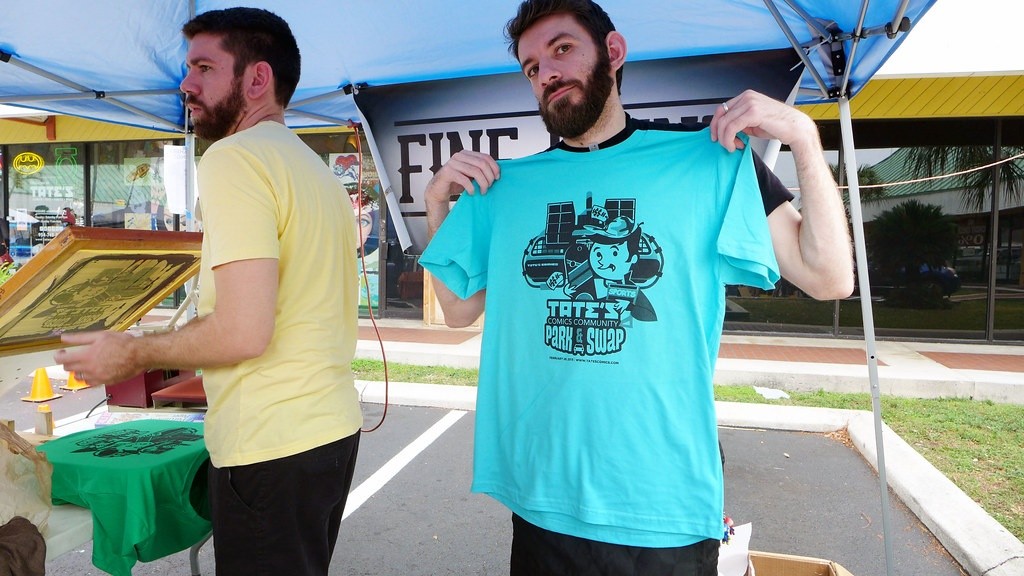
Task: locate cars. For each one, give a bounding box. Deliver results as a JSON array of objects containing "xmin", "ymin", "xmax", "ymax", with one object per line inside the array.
[{"xmin": 854, "ymin": 247, "xmax": 960, "ymax": 294}]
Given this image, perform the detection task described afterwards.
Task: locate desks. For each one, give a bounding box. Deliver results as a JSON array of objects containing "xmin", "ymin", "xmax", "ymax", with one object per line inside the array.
[{"xmin": 1, "ymin": 406, "xmax": 210, "ymax": 576}]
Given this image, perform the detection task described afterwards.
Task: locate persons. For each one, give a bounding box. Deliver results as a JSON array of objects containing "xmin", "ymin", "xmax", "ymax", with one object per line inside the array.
[
  {"xmin": 423, "ymin": 1, "xmax": 855, "ymax": 575},
  {"xmin": 51, "ymin": 7, "xmax": 363, "ymax": 576}
]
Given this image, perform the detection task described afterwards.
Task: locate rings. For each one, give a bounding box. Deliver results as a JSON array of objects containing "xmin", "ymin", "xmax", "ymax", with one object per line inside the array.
[
  {"xmin": 80, "ymin": 373, "xmax": 84, "ymax": 381},
  {"xmin": 722, "ymin": 102, "xmax": 729, "ymax": 114}
]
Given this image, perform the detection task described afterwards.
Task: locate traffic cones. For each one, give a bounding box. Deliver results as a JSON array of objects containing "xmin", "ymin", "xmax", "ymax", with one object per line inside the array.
[
  {"xmin": 21, "ymin": 367, "xmax": 63, "ymax": 402},
  {"xmin": 60, "ymin": 370, "xmax": 91, "ymax": 391}
]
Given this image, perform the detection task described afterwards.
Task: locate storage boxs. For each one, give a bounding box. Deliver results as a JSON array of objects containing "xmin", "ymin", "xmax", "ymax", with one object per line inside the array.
[{"xmin": 745, "ymin": 550, "xmax": 855, "ymax": 576}]
